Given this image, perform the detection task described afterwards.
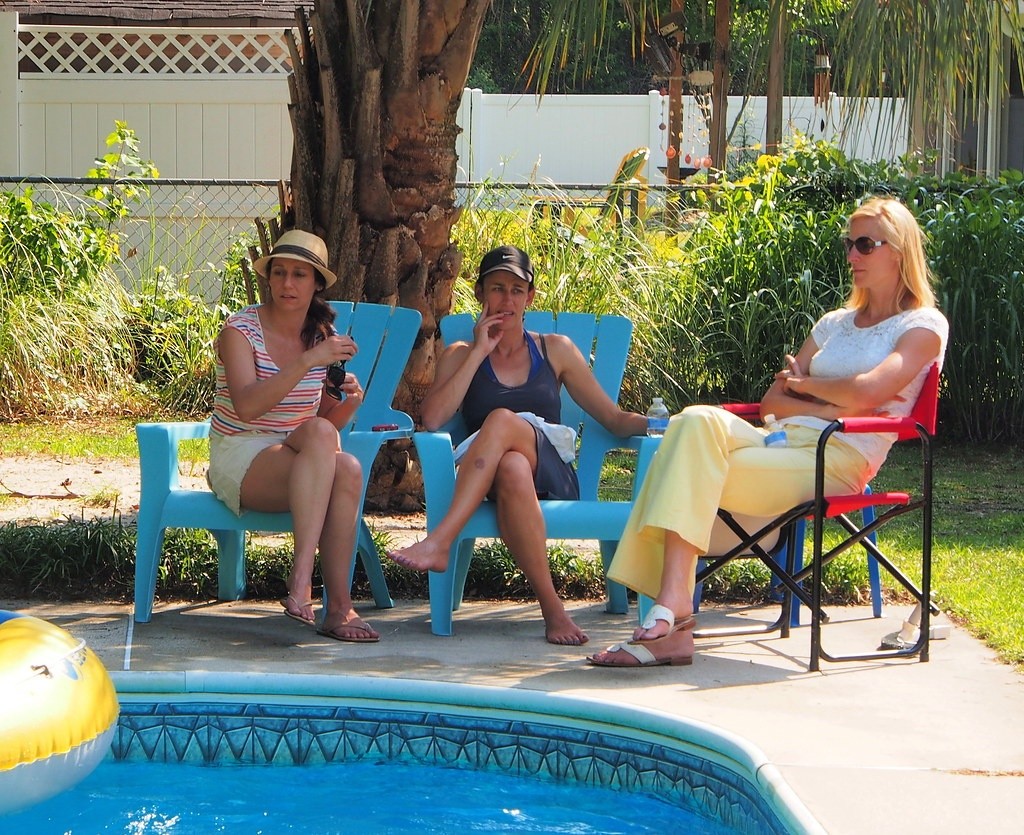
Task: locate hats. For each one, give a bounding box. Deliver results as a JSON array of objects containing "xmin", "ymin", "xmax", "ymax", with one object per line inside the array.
[
  {"xmin": 253, "ymin": 230, "xmax": 338, "ymax": 289},
  {"xmin": 479, "ymin": 246, "xmax": 535, "ymax": 285}
]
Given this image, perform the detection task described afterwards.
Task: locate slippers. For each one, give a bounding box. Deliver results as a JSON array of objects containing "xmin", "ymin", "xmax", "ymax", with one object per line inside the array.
[
  {"xmin": 280, "ymin": 594, "xmax": 316, "ymax": 626},
  {"xmin": 587, "ymin": 640, "xmax": 692, "ymax": 667},
  {"xmin": 317, "ymin": 618, "xmax": 379, "ymax": 643},
  {"xmin": 627, "ymin": 604, "xmax": 697, "ymax": 646}
]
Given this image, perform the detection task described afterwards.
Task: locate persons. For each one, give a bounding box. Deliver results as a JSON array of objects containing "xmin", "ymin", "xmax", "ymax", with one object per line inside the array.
[
  {"xmin": 209, "ymin": 229, "xmax": 380, "ymax": 643},
  {"xmin": 387, "ymin": 246, "xmax": 665, "ymax": 645},
  {"xmin": 587, "ymin": 200, "xmax": 949, "ymax": 668}
]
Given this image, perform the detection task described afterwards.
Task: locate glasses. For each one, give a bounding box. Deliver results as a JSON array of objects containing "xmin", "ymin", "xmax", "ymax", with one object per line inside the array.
[
  {"xmin": 324, "ymin": 361, "xmax": 346, "ymax": 401},
  {"xmin": 844, "ymin": 236, "xmax": 887, "ymax": 254}
]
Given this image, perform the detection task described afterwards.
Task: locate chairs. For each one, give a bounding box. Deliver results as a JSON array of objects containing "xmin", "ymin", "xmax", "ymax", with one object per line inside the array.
[
  {"xmin": 514, "ymin": 147, "xmax": 650, "ymax": 235},
  {"xmin": 693, "ymin": 360, "xmax": 939, "ymax": 672},
  {"xmin": 414, "ymin": 311, "xmax": 665, "ymax": 636},
  {"xmin": 135, "ymin": 301, "xmax": 420, "ymax": 622}
]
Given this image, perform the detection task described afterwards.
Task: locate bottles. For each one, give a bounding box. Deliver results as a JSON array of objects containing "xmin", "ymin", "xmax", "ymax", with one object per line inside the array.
[
  {"xmin": 646, "ymin": 397, "xmax": 670, "ymax": 438},
  {"xmin": 762, "ymin": 413, "xmax": 788, "ymax": 448}
]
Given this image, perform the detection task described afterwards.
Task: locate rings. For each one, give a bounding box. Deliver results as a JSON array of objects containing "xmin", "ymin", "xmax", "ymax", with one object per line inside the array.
[{"xmin": 353, "ymin": 392, "xmax": 358, "ymax": 399}]
